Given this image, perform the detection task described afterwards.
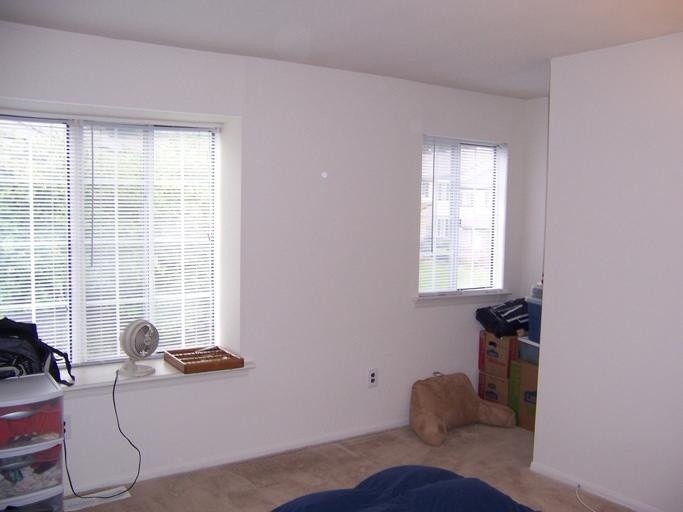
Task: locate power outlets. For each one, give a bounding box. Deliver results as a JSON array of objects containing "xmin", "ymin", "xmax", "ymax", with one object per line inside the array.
[
  {"xmin": 368, "ymin": 368, "xmax": 378, "ymax": 386},
  {"xmin": 63, "ymin": 415, "xmax": 71, "ymax": 439}
]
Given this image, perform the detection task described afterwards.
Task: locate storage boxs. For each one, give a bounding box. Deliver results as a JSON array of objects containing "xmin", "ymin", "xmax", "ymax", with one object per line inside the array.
[{"xmin": 479, "ymin": 286, "xmax": 543, "ymax": 431}]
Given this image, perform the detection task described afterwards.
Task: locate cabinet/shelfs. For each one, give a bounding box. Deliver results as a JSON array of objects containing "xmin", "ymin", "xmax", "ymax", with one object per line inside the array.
[{"xmin": 0, "ymin": 372, "xmax": 64, "ymax": 512}]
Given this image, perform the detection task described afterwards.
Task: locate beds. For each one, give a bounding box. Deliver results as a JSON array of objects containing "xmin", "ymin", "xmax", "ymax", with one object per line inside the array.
[{"xmin": 270, "ymin": 466, "xmax": 535, "ymax": 512}]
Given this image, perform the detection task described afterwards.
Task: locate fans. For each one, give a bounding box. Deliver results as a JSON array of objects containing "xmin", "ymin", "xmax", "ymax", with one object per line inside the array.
[{"xmin": 118, "ymin": 320, "xmax": 159, "ymax": 377}]
